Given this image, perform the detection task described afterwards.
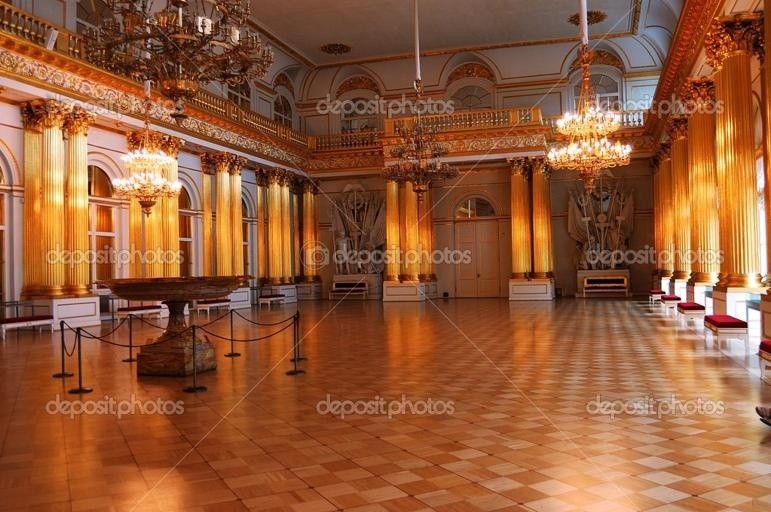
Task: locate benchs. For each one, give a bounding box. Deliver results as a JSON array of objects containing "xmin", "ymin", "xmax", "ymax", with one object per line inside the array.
[
  {"xmin": 647, "ymin": 288, "xmax": 771, "ymax": 371},
  {"xmin": 328, "ymin": 281, "xmax": 368, "ymax": 301},
  {"xmin": 116, "ymin": 305, "xmax": 161, "ymax": 324},
  {"xmin": 582, "ymin": 276, "xmax": 628, "ymax": 299},
  {"xmin": 196, "ymin": 299, "xmax": 230, "ymax": 316},
  {"xmin": 258, "ymin": 294, "xmax": 284, "ymax": 308},
  {"xmin": 0, "ymin": 315, "xmax": 53, "ymax": 340}
]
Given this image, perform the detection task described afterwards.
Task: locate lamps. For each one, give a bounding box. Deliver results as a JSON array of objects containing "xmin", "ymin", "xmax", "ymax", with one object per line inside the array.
[
  {"xmin": 377, "ymin": 0, "xmax": 458, "ymax": 202},
  {"xmin": 110, "ymin": 112, "xmax": 185, "ymax": 219},
  {"xmin": 80, "ymin": 0, "xmax": 274, "ymax": 127},
  {"xmin": 546, "ymin": 0, "xmax": 632, "ymax": 194}
]
